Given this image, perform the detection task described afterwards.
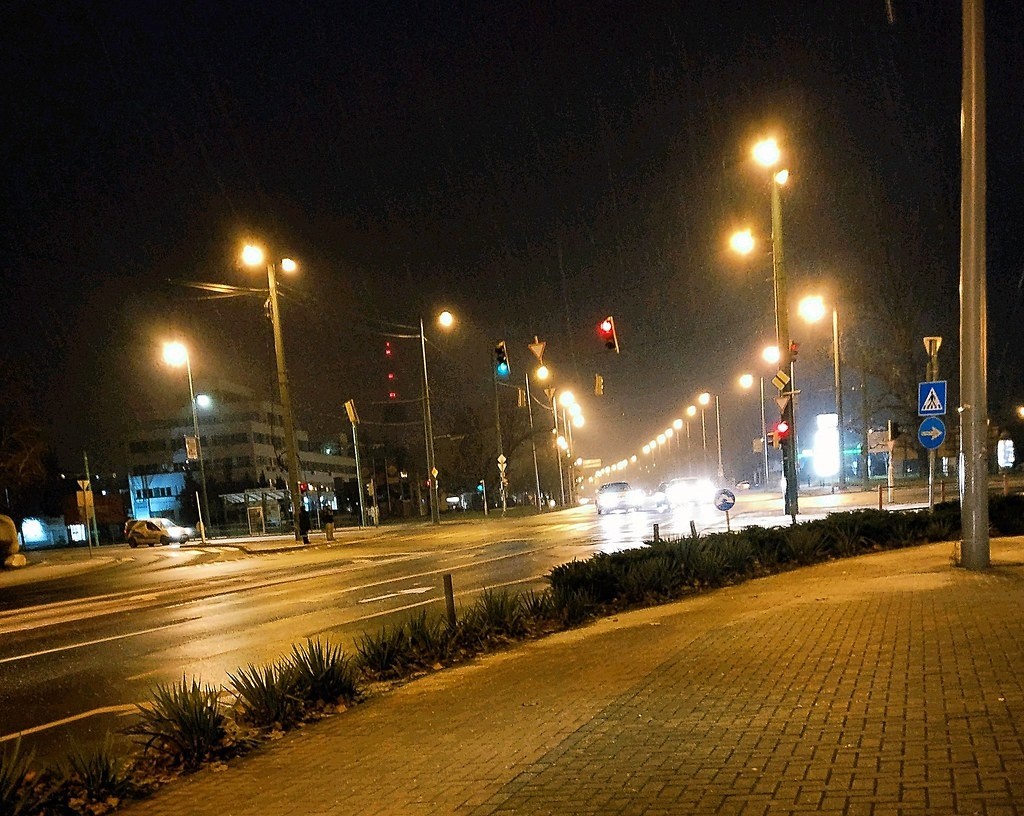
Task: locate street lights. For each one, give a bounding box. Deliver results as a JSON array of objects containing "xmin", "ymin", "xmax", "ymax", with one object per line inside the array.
[
  {"xmin": 798, "ymin": 298, "xmax": 852, "ymax": 491},
  {"xmin": 417, "ymin": 311, "xmax": 452, "ymax": 527},
  {"xmin": 753, "ymin": 137, "xmax": 805, "ymax": 520},
  {"xmin": 740, "ymin": 372, "xmax": 771, "ymax": 489},
  {"xmin": 699, "ymin": 393, "xmax": 727, "ymax": 488},
  {"xmin": 686, "ymin": 405, "xmax": 710, "ymax": 478},
  {"xmin": 237, "ymin": 242, "xmax": 306, "ymax": 543},
  {"xmin": 618, "ymin": 419, "xmax": 684, "ymax": 483},
  {"xmin": 523, "ymin": 363, "xmax": 550, "ymax": 511},
  {"xmin": 550, "ymin": 390, "xmax": 586, "ymax": 508},
  {"xmin": 162, "ymin": 342, "xmax": 215, "ymax": 540}
]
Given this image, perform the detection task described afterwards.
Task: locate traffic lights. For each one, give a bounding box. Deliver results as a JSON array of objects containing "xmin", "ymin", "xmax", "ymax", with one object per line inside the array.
[
  {"xmin": 599, "ymin": 315, "xmax": 622, "ymax": 355},
  {"xmin": 494, "ymin": 341, "xmax": 511, "ymax": 378},
  {"xmin": 778, "ymin": 421, "xmax": 788, "ymax": 436},
  {"xmin": 789, "ymin": 339, "xmax": 799, "ymax": 363},
  {"xmin": 765, "ymin": 430, "xmax": 780, "ymax": 448}
]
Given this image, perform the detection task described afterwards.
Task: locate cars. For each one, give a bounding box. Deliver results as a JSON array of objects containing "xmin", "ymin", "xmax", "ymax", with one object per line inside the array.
[
  {"xmin": 653, "ymin": 477, "xmax": 719, "ymax": 510},
  {"xmin": 594, "ymin": 481, "xmax": 639, "ymax": 515}
]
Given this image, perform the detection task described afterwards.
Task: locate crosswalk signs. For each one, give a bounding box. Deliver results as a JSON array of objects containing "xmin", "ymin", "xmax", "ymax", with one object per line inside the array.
[{"xmin": 917, "ymin": 381, "xmax": 947, "ymax": 415}]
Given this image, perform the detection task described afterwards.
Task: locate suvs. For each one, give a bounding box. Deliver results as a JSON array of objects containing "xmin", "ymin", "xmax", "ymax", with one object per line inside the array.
[{"xmin": 125, "ymin": 518, "xmax": 196, "ymax": 548}]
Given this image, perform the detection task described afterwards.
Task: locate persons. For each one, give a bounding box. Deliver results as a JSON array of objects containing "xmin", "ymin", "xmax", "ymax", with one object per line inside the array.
[
  {"xmin": 808, "ymin": 475, "xmax": 812, "ymax": 487},
  {"xmin": 322, "ymin": 505, "xmax": 336, "ymax": 540},
  {"xmin": 299, "ymin": 506, "xmax": 312, "ymax": 544}
]
